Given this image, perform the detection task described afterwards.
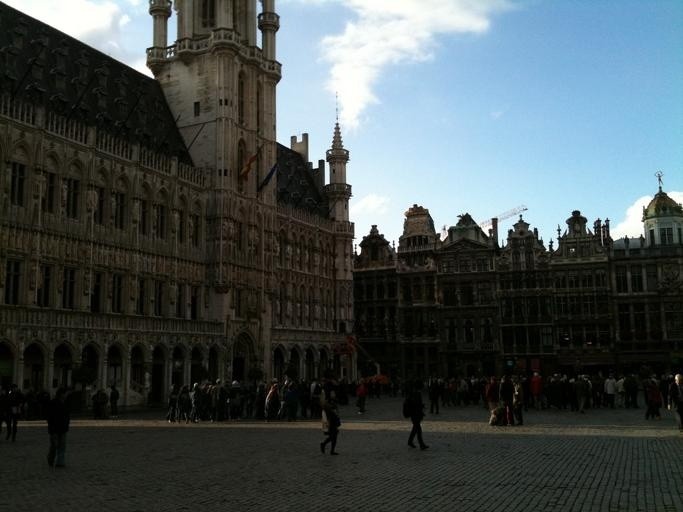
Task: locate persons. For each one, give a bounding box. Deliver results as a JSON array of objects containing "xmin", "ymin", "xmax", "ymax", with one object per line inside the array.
[
  {"xmin": 0, "ymin": 383, "xmax": 119, "ymax": 467},
  {"xmin": 166, "ymin": 373, "xmax": 682, "ymax": 434},
  {"xmin": 320, "ymin": 397, "xmax": 341, "ymax": 455},
  {"xmin": 407, "ymin": 380, "xmax": 428, "ymax": 449}
]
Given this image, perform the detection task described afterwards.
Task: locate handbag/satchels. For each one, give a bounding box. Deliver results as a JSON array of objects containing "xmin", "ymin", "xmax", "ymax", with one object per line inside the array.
[{"xmin": 12, "ymin": 407, "xmax": 22, "ymax": 415}]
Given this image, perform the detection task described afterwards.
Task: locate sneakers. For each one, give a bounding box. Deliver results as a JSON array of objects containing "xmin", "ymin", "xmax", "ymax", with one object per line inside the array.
[
  {"xmin": 408, "ymin": 442, "xmax": 416, "ymax": 448},
  {"xmin": 420, "ymin": 443, "xmax": 429, "ymax": 449},
  {"xmin": 5, "ymin": 433, "xmax": 16, "ymax": 442},
  {"xmin": 320, "ymin": 442, "xmax": 340, "ymax": 455},
  {"xmin": 47, "ymin": 456, "xmax": 65, "ymax": 468}
]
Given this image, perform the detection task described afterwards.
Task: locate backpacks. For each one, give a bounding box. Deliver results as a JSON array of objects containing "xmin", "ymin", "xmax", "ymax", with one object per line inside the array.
[{"xmin": 403, "ymin": 398, "xmax": 411, "ymax": 418}]
